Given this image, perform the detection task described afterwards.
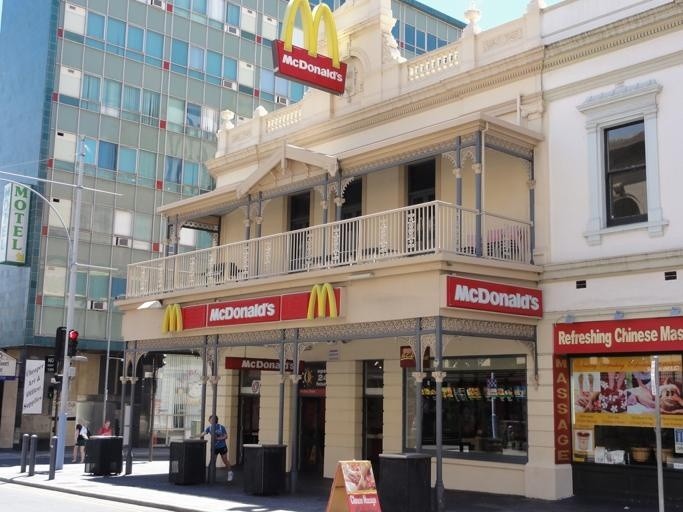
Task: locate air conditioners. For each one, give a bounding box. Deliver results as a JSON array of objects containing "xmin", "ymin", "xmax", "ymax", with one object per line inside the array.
[
  {"xmin": 115, "ymin": 236, "xmax": 129, "ymax": 248},
  {"xmin": 223, "ymin": 80, "xmax": 232, "ymax": 89},
  {"xmin": 227, "ymin": 25, "xmax": 240, "ymax": 37},
  {"xmin": 151, "ymin": 0, "xmax": 164, "ymax": 9},
  {"xmin": 90, "ymin": 299, "xmax": 107, "ymax": 311},
  {"xmin": 276, "ymin": 96, "xmax": 287, "ymax": 106}
]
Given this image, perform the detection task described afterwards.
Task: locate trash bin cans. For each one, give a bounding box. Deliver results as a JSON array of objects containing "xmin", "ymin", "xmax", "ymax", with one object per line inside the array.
[
  {"xmin": 169, "ymin": 439, "xmax": 208, "ymax": 486},
  {"xmin": 378, "ymin": 452, "xmax": 432, "ymax": 512},
  {"xmin": 242, "ymin": 444, "xmax": 288, "ymax": 496},
  {"xmin": 85, "ymin": 435, "xmax": 124, "ymax": 476}
]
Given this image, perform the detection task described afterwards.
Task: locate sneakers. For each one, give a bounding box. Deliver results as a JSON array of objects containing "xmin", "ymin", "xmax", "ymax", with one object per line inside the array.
[{"xmin": 227, "ymin": 471, "xmax": 234, "ymax": 481}]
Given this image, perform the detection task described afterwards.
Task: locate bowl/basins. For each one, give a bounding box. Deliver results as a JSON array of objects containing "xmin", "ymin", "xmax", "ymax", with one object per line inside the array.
[
  {"xmin": 631, "ymin": 447, "xmax": 651, "ymax": 461},
  {"xmin": 653, "ymin": 447, "xmax": 674, "ymax": 463}
]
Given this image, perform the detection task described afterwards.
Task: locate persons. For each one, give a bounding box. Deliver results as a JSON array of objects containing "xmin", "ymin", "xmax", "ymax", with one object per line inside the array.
[
  {"xmin": 506, "ymin": 426, "xmax": 515, "ymax": 449},
  {"xmin": 189, "ymin": 415, "xmax": 234, "ymax": 482},
  {"xmin": 71, "ymin": 424, "xmax": 88, "ymax": 464},
  {"xmin": 575, "ymin": 371, "xmax": 683, "ymax": 415},
  {"xmin": 96, "ymin": 419, "xmax": 113, "ymax": 436}
]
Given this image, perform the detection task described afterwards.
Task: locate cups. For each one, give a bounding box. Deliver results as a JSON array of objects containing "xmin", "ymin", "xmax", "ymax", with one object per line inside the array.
[{"xmin": 576, "ymin": 431, "xmax": 590, "ymax": 450}]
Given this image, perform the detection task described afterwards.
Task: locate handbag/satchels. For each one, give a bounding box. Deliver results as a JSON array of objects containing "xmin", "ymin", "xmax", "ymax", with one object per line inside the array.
[{"xmin": 87, "ymin": 429, "xmax": 91, "ymax": 438}]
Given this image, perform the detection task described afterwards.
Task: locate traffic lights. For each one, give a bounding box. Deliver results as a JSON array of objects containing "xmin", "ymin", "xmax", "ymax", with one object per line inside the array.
[{"xmin": 68, "ymin": 330, "xmax": 79, "ymax": 357}]
